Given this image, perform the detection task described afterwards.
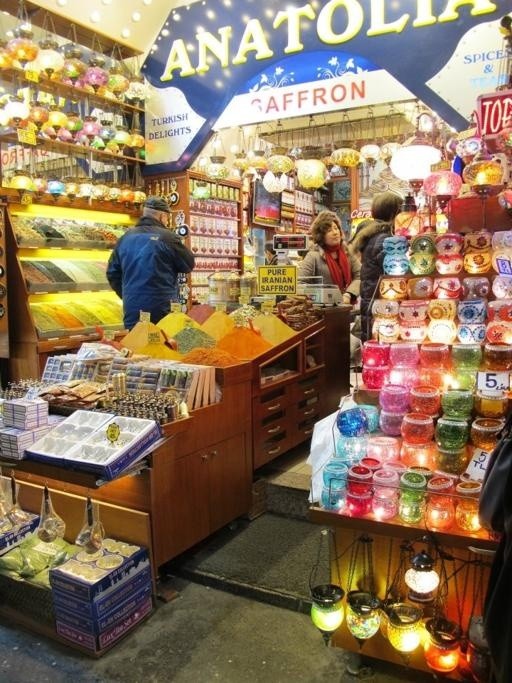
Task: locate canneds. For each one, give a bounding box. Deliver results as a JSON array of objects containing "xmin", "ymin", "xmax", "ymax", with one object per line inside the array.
[{"xmin": 112, "ymin": 372, "xmax": 126, "ymax": 396}]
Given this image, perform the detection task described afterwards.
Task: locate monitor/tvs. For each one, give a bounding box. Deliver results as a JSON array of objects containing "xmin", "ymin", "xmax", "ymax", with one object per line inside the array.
[{"xmin": 251, "ymin": 178, "xmax": 282, "ymax": 227}]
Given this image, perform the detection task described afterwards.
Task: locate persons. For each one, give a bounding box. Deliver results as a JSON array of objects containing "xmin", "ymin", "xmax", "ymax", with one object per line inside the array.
[
  {"xmin": 296, "ymin": 208, "xmax": 363, "ymax": 309},
  {"xmin": 477, "ymin": 397, "xmax": 512, "ymax": 682},
  {"xmin": 263, "ymin": 244, "xmax": 278, "ymax": 265},
  {"xmin": 344, "ymin": 191, "xmax": 404, "ymax": 344},
  {"xmin": 105, "ymin": 193, "xmax": 196, "ymax": 332}
]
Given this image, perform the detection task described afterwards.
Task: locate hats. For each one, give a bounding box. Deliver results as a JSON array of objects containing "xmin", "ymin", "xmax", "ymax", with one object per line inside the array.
[{"xmin": 143, "ymin": 196, "xmax": 173, "ymax": 213}]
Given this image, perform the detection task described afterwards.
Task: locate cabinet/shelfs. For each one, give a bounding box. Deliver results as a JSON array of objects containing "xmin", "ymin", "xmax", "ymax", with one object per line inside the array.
[
  {"xmin": 306, "ymin": 151, "xmax": 512, "ymax": 680},
  {"xmin": 0, "ymin": 0, "xmax": 355, "ymax": 568}
]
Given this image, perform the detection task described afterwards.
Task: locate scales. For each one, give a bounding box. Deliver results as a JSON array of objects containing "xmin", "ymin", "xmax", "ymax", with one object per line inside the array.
[{"xmin": 273, "ymin": 234, "xmax": 344, "ymax": 307}]
